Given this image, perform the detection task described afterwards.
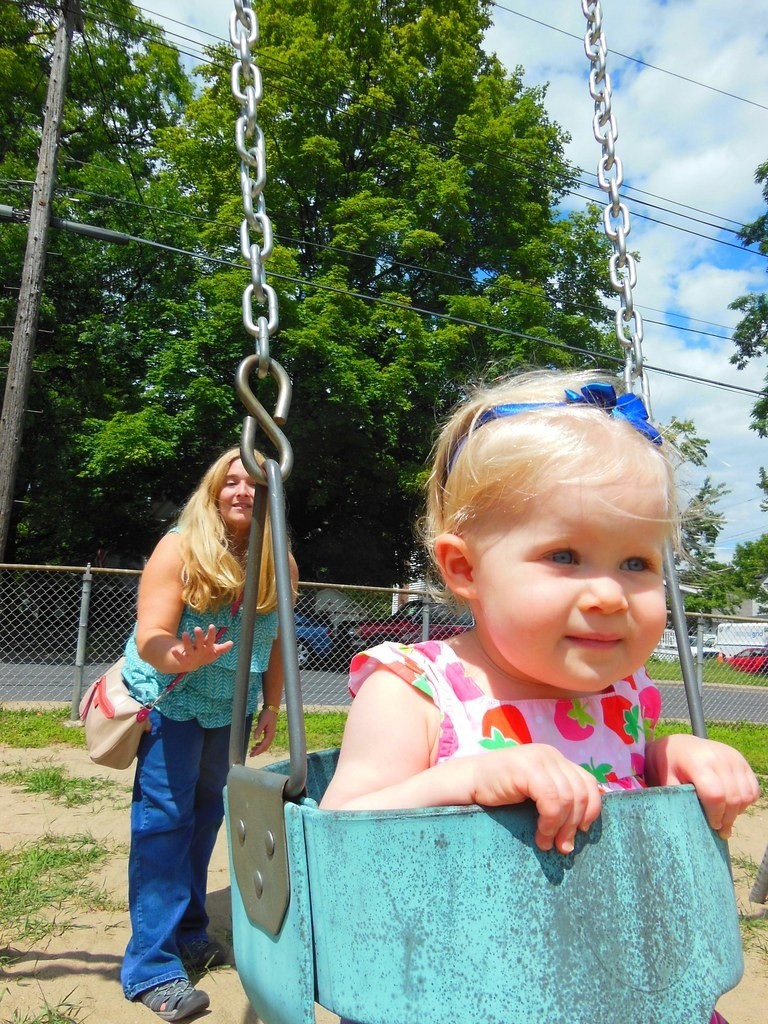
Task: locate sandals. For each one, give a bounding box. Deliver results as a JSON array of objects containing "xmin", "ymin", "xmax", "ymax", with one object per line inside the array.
[
  {"xmin": 139, "ymin": 978, "xmax": 209, "ymax": 1022},
  {"xmin": 182, "ymin": 940, "xmax": 227, "ymax": 973}
]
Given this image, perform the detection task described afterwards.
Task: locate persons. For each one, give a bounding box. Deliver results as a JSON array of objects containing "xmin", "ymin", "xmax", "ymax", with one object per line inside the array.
[
  {"xmin": 318, "ymin": 366, "xmax": 761, "ymax": 1024},
  {"xmin": 120, "ymin": 447, "xmax": 298, "ymax": 1022}
]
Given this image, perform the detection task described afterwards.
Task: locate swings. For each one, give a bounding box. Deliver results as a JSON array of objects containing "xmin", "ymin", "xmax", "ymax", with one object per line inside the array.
[{"xmin": 218, "ymin": 0, "xmax": 744, "ymax": 1024}]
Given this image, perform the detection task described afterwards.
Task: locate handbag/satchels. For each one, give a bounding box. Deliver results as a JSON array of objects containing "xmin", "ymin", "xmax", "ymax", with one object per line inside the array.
[{"xmin": 80, "ymin": 658, "xmax": 156, "ymax": 771}]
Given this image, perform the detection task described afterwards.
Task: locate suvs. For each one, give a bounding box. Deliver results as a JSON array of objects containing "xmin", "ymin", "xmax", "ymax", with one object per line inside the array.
[{"xmin": 338, "ymin": 599, "xmax": 476, "ymax": 673}]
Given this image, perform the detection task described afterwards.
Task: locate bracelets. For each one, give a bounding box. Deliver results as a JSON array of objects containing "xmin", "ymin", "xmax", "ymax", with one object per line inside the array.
[{"xmin": 262, "ymin": 704, "xmax": 279, "ymax": 714}]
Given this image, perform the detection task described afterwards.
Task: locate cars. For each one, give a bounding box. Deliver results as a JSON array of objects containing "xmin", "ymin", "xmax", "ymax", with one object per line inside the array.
[
  {"xmin": 650, "ymin": 619, "xmax": 768, "ymax": 679},
  {"xmin": 291, "ymin": 607, "xmax": 335, "ymax": 671}
]
